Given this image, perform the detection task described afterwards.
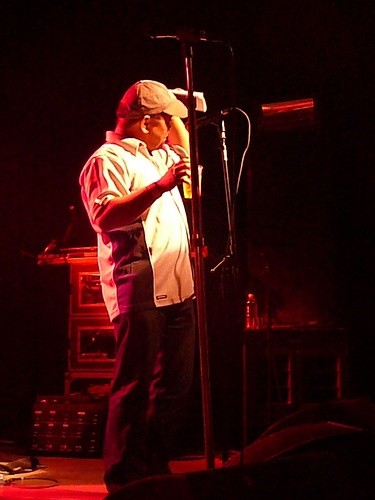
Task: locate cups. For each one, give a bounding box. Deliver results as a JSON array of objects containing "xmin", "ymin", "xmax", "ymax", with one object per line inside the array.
[{"xmin": 182, "ymin": 164, "xmax": 205, "ymax": 199}]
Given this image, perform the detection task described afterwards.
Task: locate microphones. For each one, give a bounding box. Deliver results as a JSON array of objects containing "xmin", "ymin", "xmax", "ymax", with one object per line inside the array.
[{"xmin": 185, "ymin": 107, "xmax": 234, "ymax": 132}]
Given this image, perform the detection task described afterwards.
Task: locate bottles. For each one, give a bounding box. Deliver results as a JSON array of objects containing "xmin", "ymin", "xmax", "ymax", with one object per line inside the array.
[{"xmin": 246, "ymin": 293, "xmax": 258, "ymax": 329}]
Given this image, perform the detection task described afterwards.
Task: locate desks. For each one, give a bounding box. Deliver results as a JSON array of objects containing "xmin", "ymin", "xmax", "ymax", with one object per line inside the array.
[{"xmin": 240, "ymin": 324, "xmax": 348, "ymax": 446}]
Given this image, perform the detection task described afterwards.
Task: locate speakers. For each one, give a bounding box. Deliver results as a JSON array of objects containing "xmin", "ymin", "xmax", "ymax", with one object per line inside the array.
[{"xmin": 103, "ymin": 417, "xmax": 375, "ymax": 500}]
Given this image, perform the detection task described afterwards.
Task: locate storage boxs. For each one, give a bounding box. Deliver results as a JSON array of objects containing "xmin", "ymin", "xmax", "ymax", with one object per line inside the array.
[{"xmin": 64, "ymin": 252, "xmax": 118, "ymax": 393}]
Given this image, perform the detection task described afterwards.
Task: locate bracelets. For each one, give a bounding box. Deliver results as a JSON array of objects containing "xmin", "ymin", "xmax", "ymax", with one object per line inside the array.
[{"xmin": 150, "ymin": 183, "xmax": 162, "ymax": 198}]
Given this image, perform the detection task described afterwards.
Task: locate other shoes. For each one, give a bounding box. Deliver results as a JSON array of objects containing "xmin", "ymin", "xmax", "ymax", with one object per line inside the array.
[
  {"xmin": 146, "ymin": 460, "xmax": 173, "ymax": 476},
  {"xmin": 105, "ymin": 468, "xmax": 145, "ymax": 494}
]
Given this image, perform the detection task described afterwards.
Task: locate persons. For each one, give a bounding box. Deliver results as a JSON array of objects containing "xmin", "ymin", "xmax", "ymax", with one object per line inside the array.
[{"xmin": 78, "ymin": 79, "xmax": 199, "ymax": 491}]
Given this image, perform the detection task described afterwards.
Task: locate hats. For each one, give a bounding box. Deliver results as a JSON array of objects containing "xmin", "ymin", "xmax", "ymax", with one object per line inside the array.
[{"xmin": 115, "ymin": 78, "xmax": 189, "ymax": 120}]
